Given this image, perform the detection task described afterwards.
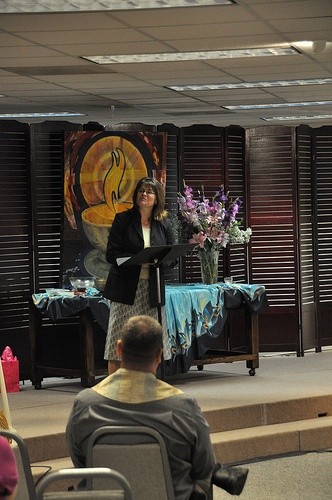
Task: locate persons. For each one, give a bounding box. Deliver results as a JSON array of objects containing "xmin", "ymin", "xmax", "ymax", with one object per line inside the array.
[
  {"xmin": 65, "ymin": 315, "xmax": 249, "ymax": 500},
  {"xmin": 0, "ymin": 435, "xmax": 18, "ymax": 500},
  {"xmin": 104, "ymin": 178, "xmax": 179, "ymax": 378}
]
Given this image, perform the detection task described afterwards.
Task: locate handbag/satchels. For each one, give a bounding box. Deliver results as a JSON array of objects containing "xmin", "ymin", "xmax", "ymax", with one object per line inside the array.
[{"xmin": 0, "ymin": 346, "xmax": 20, "ymax": 393}]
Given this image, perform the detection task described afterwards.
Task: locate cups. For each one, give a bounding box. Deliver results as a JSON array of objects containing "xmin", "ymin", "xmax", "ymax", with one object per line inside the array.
[
  {"xmin": 69, "ymin": 277, "xmax": 96, "ymax": 292},
  {"xmin": 224, "ymin": 276, "xmax": 233, "ymax": 286},
  {"xmin": 45, "ymin": 288, "xmax": 63, "ymax": 296}
]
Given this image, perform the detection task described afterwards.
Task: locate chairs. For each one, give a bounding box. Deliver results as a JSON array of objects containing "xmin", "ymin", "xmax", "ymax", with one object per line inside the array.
[{"xmin": 0, "ymin": 421, "xmax": 175, "ymax": 500}]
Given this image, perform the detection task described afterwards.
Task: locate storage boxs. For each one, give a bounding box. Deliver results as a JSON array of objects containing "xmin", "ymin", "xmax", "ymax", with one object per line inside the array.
[{"xmin": 2, "ymin": 359, "xmax": 20, "ymax": 392}]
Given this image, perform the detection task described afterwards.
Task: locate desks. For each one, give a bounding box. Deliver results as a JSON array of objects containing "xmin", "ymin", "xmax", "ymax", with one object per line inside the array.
[{"xmin": 27, "ymin": 281, "xmax": 266, "ymax": 390}]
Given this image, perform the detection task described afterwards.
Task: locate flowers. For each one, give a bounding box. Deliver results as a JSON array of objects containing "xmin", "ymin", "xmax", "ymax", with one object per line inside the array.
[{"xmin": 178, "ymin": 183, "xmax": 251, "ymax": 246}]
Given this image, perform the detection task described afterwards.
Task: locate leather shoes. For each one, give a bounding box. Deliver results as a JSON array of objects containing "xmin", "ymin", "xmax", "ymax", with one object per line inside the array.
[{"xmin": 213, "ymin": 464, "xmax": 249, "ymax": 496}]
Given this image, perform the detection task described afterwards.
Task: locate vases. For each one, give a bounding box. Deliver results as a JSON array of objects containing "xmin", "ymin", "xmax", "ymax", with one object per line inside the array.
[{"xmin": 199, "ymin": 248, "xmax": 223, "ymax": 284}]
